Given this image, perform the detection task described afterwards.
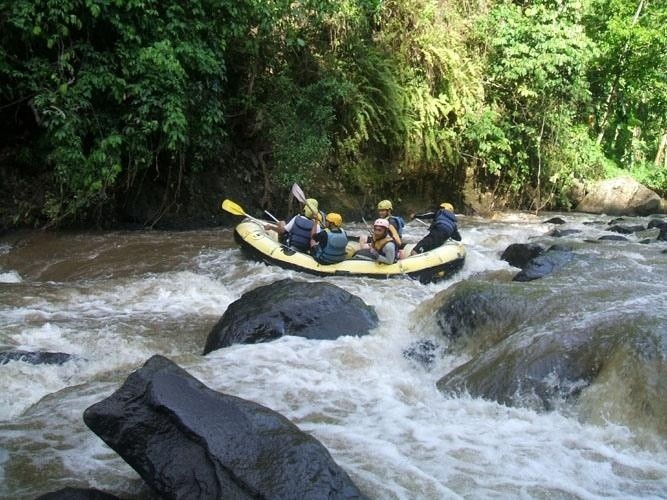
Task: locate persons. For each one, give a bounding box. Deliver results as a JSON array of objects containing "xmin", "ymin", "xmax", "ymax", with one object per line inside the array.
[
  {"xmin": 265, "ymin": 198, "xmax": 326, "ymax": 255},
  {"xmin": 377, "ymin": 199, "xmax": 405, "ymax": 239},
  {"xmin": 410, "ymin": 202, "xmax": 461, "ymax": 255},
  {"xmin": 352, "ymin": 219, "xmax": 401, "ymax": 265},
  {"xmin": 309, "ymin": 212, "xmax": 349, "ymax": 264}
]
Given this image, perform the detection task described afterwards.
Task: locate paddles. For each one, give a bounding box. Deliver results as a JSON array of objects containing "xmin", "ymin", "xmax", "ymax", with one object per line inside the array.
[
  {"xmin": 221, "ymin": 199, "xmax": 264, "ymax": 227},
  {"xmin": 252, "ymin": 199, "xmax": 279, "ymax": 222},
  {"xmin": 291, "ymin": 183, "xmax": 325, "ymax": 228}
]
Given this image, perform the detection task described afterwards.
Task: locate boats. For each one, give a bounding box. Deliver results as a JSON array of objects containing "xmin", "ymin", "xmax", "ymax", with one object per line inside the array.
[{"xmin": 234, "ymin": 218, "xmax": 467, "ymax": 284}]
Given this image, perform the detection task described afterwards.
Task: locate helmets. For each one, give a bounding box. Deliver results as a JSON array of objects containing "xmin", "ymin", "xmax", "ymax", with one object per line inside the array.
[
  {"xmin": 440, "ymin": 203, "xmax": 454, "ymax": 212},
  {"xmin": 303, "ymin": 198, "xmax": 322, "ymax": 224},
  {"xmin": 377, "ymin": 199, "xmax": 393, "ymax": 210},
  {"xmin": 325, "ymin": 213, "xmax": 343, "ymax": 227},
  {"xmin": 374, "ymin": 218, "xmax": 390, "ymax": 229}
]
